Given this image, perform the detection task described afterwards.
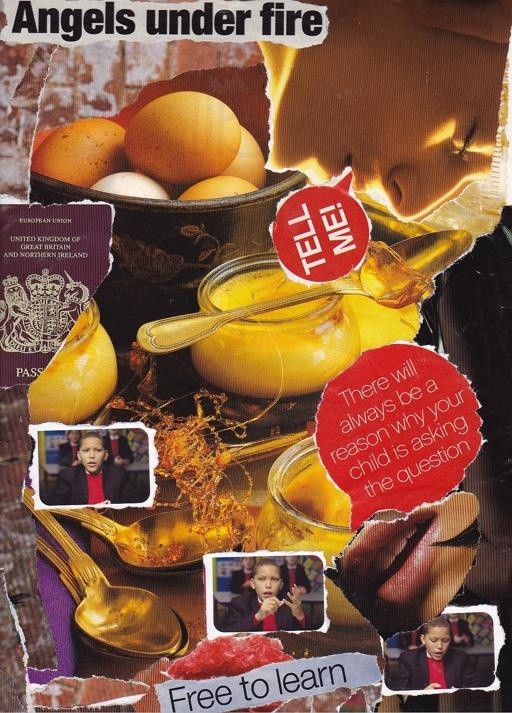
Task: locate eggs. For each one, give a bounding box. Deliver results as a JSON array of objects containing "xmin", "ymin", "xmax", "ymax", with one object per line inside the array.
[{"xmin": 32, "ymin": 91, "xmax": 268, "ymax": 202}]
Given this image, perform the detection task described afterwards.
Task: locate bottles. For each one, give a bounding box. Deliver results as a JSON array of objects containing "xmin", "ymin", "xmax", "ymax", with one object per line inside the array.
[
  {"xmin": 28, "ymin": 295, "xmax": 119, "ymax": 427},
  {"xmin": 253, "ymin": 431, "xmax": 379, "ymax": 630},
  {"xmin": 190, "ymin": 247, "xmax": 363, "ymax": 401}
]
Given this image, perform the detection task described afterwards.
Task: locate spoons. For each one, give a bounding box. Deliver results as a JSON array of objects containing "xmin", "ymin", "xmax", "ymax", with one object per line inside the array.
[
  {"xmin": 135, "ymin": 225, "xmax": 480, "ymax": 359},
  {"xmin": 18, "ymin": 485, "xmax": 246, "ymax": 666}
]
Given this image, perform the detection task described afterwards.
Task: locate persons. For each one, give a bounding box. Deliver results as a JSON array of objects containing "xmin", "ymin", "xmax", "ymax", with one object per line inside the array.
[
  {"xmin": 275, "ymin": 0, "xmax": 511, "ymax": 220},
  {"xmin": 339, "ymin": 491, "xmax": 480, "ymax": 627},
  {"xmin": 224, "ymin": 554, "xmax": 313, "ymax": 632},
  {"xmin": 38, "ymin": 427, "xmax": 141, "ymax": 504},
  {"xmin": 382, "ymin": 612, "xmax": 494, "ymax": 694}
]
